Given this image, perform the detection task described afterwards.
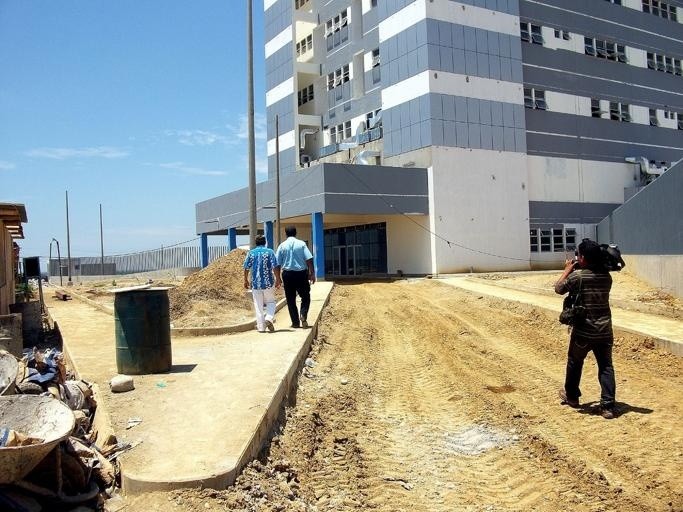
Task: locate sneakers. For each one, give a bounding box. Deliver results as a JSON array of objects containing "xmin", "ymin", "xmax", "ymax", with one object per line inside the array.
[{"xmin": 264, "ymin": 320, "xmax": 274, "ymax": 332}]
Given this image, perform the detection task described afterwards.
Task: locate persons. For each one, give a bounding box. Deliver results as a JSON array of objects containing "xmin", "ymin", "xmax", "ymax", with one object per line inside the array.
[
  {"xmin": 555, "ymin": 240, "xmax": 615, "ymax": 419},
  {"xmin": 275, "ymin": 226, "xmax": 316, "ymax": 327},
  {"xmin": 244, "ymin": 235, "xmax": 283, "ymax": 333}
]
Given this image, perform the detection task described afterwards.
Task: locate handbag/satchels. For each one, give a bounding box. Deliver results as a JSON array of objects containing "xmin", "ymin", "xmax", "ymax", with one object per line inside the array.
[{"xmin": 558, "ymin": 271, "xmax": 586, "ymax": 324}]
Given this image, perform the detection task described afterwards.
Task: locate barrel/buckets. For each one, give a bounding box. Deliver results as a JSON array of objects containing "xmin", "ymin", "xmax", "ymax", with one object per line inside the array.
[
  {"xmin": 8, "ymin": 300, "xmax": 45, "ymax": 348},
  {"xmin": 114, "ymin": 286, "xmax": 175, "ymax": 376}
]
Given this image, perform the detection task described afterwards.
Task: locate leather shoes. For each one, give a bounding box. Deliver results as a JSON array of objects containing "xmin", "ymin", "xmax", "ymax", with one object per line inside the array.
[
  {"xmin": 594, "ymin": 404, "xmax": 613, "ymax": 418},
  {"xmin": 558, "ymin": 389, "xmax": 579, "ymax": 407},
  {"xmin": 299, "ymin": 313, "xmax": 307, "ymax": 328},
  {"xmin": 291, "ymin": 323, "xmax": 299, "ymax": 328}
]
